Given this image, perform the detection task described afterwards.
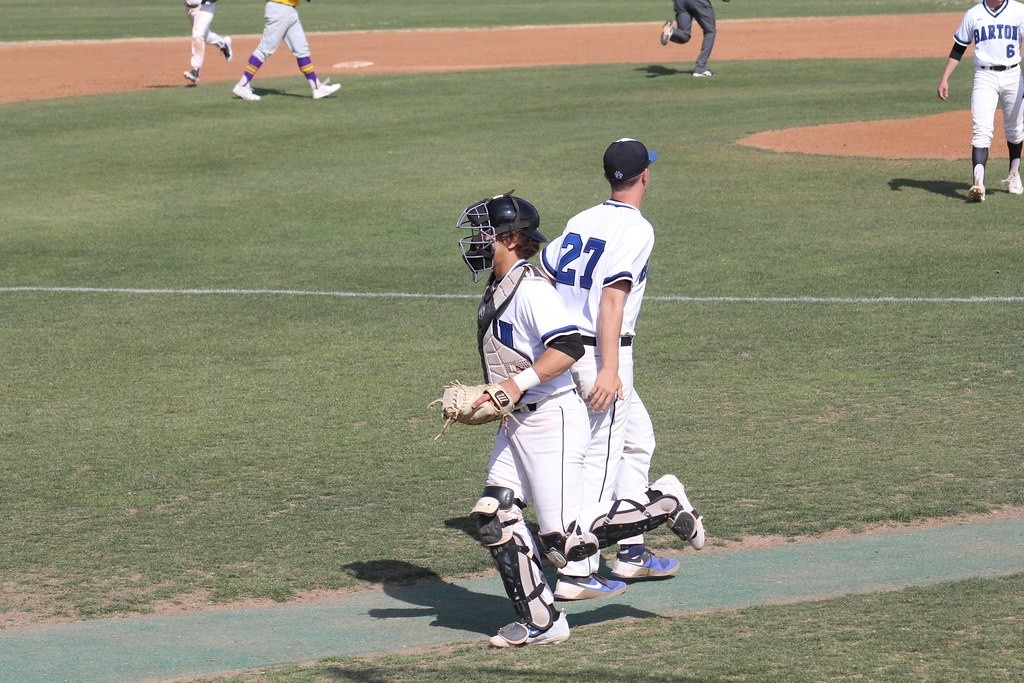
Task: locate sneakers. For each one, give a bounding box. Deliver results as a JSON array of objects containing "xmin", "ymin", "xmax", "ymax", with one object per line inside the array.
[
  {"xmin": 649, "ymin": 473, "xmax": 704, "ymax": 549},
  {"xmin": 312, "ymin": 83, "xmax": 341, "ymax": 98},
  {"xmin": 968, "ymin": 182, "xmax": 985, "ymax": 201},
  {"xmin": 232, "ymin": 83, "xmax": 261, "ymax": 99},
  {"xmin": 611, "ymin": 544, "xmax": 681, "ymax": 578},
  {"xmin": 553, "ymin": 573, "xmax": 626, "ymax": 599},
  {"xmin": 184, "ymin": 69, "xmax": 200, "ymax": 83},
  {"xmin": 489, "ymin": 608, "xmax": 570, "ymax": 647},
  {"xmin": 1002, "ymin": 171, "xmax": 1023, "ymax": 195},
  {"xmin": 220, "ymin": 35, "xmax": 232, "ymax": 61}
]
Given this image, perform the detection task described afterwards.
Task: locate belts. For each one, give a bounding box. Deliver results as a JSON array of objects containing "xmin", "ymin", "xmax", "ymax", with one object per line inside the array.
[
  {"xmin": 582, "ymin": 335, "xmax": 631, "ymax": 346},
  {"xmin": 513, "ymin": 389, "xmax": 576, "ymax": 412},
  {"xmin": 981, "ymin": 63, "xmax": 1018, "ymax": 70}
]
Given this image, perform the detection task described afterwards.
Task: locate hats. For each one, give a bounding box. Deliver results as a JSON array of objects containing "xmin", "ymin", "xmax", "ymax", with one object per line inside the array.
[{"xmin": 603, "ymin": 137, "xmax": 657, "ymax": 181}]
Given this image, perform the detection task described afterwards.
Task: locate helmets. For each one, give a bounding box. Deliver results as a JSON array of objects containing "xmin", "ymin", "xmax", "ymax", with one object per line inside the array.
[{"xmin": 487, "ymin": 195, "xmax": 549, "ymax": 242}]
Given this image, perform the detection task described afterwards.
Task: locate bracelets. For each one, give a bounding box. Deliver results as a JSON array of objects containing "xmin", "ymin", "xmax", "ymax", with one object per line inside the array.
[{"xmin": 514, "ymin": 367, "xmax": 540, "ymax": 392}]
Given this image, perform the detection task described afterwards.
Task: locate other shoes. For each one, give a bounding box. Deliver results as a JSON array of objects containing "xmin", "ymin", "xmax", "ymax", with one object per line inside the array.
[
  {"xmin": 661, "ymin": 19, "xmax": 673, "ymax": 45},
  {"xmin": 693, "ymin": 69, "xmax": 714, "ymax": 76}
]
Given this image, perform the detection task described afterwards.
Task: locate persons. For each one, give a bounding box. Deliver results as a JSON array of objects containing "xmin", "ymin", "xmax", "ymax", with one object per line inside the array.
[
  {"xmin": 939, "ymin": 0, "xmax": 1024, "ymax": 202},
  {"xmin": 231, "ymin": 0, "xmax": 341, "ymax": 100},
  {"xmin": 660, "ymin": 0, "xmax": 729, "ymax": 76},
  {"xmin": 539, "ymin": 138, "xmax": 681, "ymax": 601},
  {"xmin": 183, "ymin": 0, "xmax": 233, "ymax": 83},
  {"xmin": 438, "ymin": 190, "xmax": 705, "ymax": 647}
]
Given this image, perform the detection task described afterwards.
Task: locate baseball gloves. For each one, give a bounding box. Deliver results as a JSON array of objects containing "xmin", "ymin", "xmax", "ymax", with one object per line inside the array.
[{"xmin": 424, "ymin": 377, "xmax": 522, "ymax": 443}]
